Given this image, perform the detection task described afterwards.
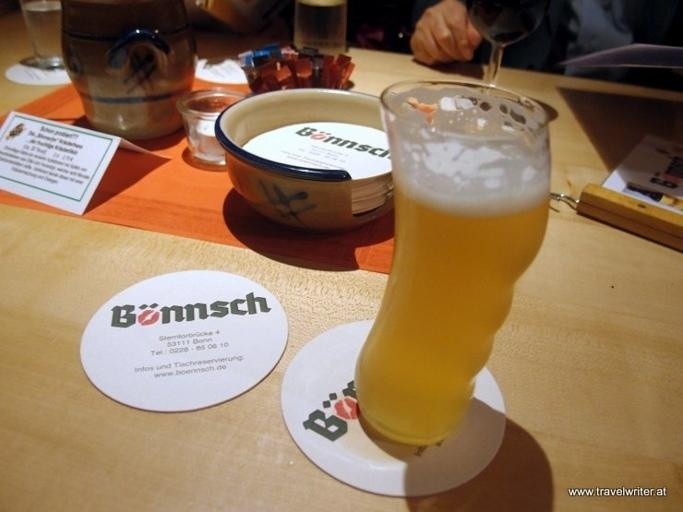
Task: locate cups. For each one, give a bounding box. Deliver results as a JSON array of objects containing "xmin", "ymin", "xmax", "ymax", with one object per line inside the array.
[
  {"xmin": 18, "ymin": 0, "xmax": 64, "ymax": 69},
  {"xmin": 294, "ymin": 0, "xmax": 348, "ymax": 55},
  {"xmin": 353, "ymin": 79, "xmax": 551, "ymax": 446}
]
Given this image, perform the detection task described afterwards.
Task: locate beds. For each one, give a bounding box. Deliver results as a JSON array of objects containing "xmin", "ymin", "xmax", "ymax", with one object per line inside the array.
[{"xmin": 1, "ymin": 8, "xmax": 682, "ymax": 510}]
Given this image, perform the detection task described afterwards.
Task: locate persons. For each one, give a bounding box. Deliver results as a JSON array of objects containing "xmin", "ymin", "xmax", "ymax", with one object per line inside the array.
[{"xmin": 410, "ymin": 0, "xmax": 683, "ymax": 80}]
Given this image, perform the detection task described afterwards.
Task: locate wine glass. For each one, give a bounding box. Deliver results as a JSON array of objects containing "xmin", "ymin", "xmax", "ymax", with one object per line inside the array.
[{"xmin": 464, "ymin": 1, "xmax": 548, "ymax": 85}]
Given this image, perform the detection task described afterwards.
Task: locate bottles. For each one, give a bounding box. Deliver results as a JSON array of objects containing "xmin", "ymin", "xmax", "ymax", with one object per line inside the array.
[{"xmin": 59, "ymin": 0, "xmax": 197, "ymax": 141}]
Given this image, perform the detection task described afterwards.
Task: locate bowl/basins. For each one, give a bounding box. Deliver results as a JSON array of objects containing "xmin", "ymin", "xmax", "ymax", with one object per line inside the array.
[
  {"xmin": 175, "ymin": 88, "xmax": 246, "ymax": 166},
  {"xmin": 214, "ymin": 87, "xmax": 394, "ymax": 234}
]
